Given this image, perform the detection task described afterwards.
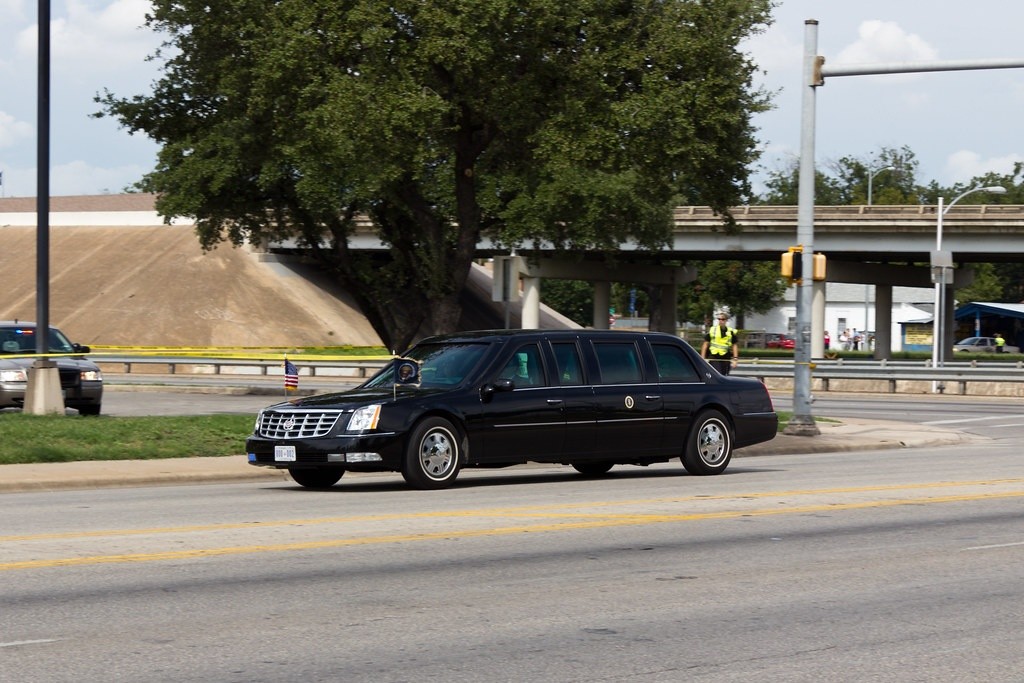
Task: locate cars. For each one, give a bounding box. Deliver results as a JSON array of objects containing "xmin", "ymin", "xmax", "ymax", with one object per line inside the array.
[
  {"xmin": 246, "ymin": 329, "xmax": 780, "ymax": 491},
  {"xmin": 953, "ymin": 336, "xmax": 1020, "ymax": 354},
  {"xmin": 0, "ymin": 321, "xmax": 102, "ymax": 417}
]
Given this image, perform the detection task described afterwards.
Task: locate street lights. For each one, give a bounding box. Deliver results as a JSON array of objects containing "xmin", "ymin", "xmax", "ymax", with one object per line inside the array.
[
  {"xmin": 932, "ymin": 186, "xmax": 1008, "ymax": 393},
  {"xmin": 864, "ymin": 167, "xmax": 897, "ymax": 351}
]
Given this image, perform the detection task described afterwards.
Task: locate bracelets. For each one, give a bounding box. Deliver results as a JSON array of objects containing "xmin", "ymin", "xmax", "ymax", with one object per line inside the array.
[{"xmin": 733, "ymin": 357, "xmax": 738, "ymax": 358}]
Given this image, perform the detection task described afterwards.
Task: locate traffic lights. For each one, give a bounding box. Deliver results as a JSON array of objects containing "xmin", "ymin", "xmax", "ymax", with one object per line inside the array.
[{"xmin": 781, "ymin": 247, "xmax": 803, "ymax": 285}]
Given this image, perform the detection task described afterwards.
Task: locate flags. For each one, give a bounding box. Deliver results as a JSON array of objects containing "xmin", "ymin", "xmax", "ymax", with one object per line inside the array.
[
  {"xmin": 393, "ymin": 355, "xmax": 422, "ymax": 387},
  {"xmin": 284, "ymin": 358, "xmax": 299, "ymax": 391}
]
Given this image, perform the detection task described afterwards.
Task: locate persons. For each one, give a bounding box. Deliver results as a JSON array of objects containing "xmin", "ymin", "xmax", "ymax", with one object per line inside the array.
[
  {"xmin": 824, "ymin": 327, "xmax": 863, "ymax": 351},
  {"xmin": 991, "ymin": 333, "xmax": 1006, "ymax": 353},
  {"xmin": 497, "ymin": 354, "xmax": 531, "ymax": 386},
  {"xmin": 701, "ymin": 311, "xmax": 739, "ymax": 376}
]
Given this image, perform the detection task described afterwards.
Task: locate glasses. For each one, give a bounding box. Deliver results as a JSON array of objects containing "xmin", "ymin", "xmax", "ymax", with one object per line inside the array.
[{"xmin": 717, "ymin": 318, "xmax": 727, "ymax": 320}]
